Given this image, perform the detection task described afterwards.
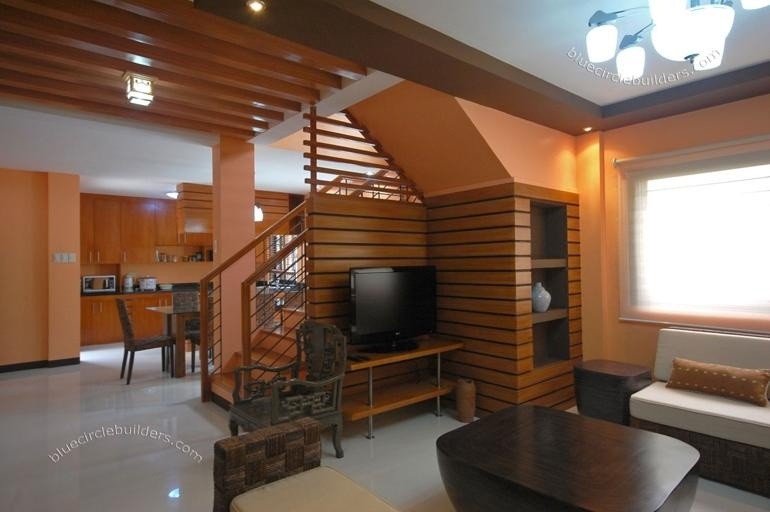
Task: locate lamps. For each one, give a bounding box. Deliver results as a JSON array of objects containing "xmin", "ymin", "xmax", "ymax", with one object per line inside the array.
[
  {"xmin": 254, "ymin": 202, "xmax": 264, "ymax": 222},
  {"xmin": 585, "ymin": 0, "xmax": 770, "ymax": 81},
  {"xmin": 121, "ymin": 69, "xmax": 160, "ymax": 107}
]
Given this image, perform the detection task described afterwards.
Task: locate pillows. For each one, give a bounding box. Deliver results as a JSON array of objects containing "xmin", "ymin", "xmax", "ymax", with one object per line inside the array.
[{"xmin": 665, "ymin": 357, "xmax": 770, "ymax": 406}]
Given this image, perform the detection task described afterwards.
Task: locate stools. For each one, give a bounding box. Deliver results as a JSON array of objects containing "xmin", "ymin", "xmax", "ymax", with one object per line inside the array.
[{"xmin": 572, "ymin": 359, "xmax": 652, "ymax": 428}]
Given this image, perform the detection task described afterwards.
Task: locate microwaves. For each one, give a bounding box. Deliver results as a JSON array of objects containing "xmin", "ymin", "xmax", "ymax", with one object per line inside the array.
[{"xmin": 82, "ymin": 275, "xmax": 116, "ymax": 295}]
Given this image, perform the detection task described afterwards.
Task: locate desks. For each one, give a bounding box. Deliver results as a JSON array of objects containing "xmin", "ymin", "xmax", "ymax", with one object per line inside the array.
[{"xmin": 145, "ymin": 306, "xmax": 210, "ymax": 378}]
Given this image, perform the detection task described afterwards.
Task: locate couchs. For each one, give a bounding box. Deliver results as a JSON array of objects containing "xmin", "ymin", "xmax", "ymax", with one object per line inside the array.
[{"xmin": 627, "ymin": 321, "xmax": 770, "ymax": 500}]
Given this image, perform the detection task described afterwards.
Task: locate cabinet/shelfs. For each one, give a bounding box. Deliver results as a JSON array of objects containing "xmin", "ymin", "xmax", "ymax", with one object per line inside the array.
[
  {"xmin": 80, "ymin": 291, "xmax": 172, "ymax": 346},
  {"xmin": 331, "ymin": 340, "xmax": 465, "ymax": 439},
  {"xmin": 80, "ymin": 193, "xmax": 178, "ymax": 265}
]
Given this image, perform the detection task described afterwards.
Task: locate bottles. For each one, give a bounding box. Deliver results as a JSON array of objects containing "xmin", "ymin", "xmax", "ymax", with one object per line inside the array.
[
  {"xmin": 122, "ymin": 275, "xmax": 135, "ymax": 294},
  {"xmin": 156, "ymin": 250, "xmax": 203, "ymax": 262}
]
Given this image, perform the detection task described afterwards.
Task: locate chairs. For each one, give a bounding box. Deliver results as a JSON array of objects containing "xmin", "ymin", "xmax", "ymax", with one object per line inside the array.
[
  {"xmin": 211, "ymin": 416, "xmax": 400, "ymax": 512},
  {"xmin": 183, "ymin": 317, "xmax": 214, "ymax": 372},
  {"xmin": 115, "ymin": 298, "xmax": 175, "ymax": 385},
  {"xmin": 228, "ymin": 321, "xmax": 347, "ymax": 459}
]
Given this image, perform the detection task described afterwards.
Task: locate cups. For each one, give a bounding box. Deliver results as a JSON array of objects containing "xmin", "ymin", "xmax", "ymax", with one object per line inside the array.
[{"xmin": 454, "ymin": 376, "xmax": 476, "ymax": 424}]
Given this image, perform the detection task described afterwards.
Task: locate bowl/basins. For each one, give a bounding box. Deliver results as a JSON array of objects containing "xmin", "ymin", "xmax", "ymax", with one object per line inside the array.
[{"xmin": 159, "ymin": 282, "xmax": 174, "ymax": 291}]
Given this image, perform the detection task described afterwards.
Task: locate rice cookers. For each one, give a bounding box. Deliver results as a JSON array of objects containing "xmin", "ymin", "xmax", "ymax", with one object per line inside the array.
[{"xmin": 138, "ymin": 275, "xmax": 158, "ymax": 292}]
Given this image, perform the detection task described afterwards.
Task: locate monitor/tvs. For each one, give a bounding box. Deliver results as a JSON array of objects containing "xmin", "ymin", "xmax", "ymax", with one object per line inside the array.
[{"xmin": 349, "ymin": 266, "xmax": 437, "ymax": 354}]
